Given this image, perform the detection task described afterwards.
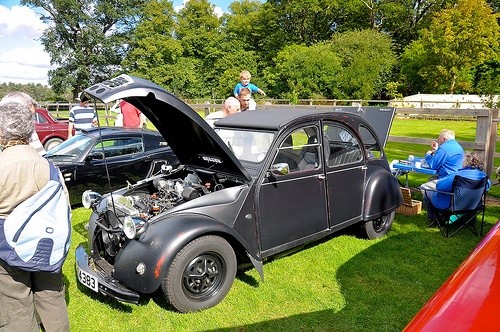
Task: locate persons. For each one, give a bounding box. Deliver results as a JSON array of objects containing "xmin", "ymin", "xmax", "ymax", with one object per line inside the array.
[
  {"xmin": 0, "ymin": 101, "xmax": 70, "ymax": 332},
  {"xmin": 68, "ymin": 93, "xmax": 98, "ymax": 141},
  {"xmin": 237, "ymin": 88, "xmax": 258, "ymax": 113},
  {"xmin": 110, "ymin": 98, "xmax": 147, "ymax": 145},
  {"xmin": 233, "ymin": 70, "xmax": 267, "ymax": 100},
  {"xmin": 424, "ymin": 151, "xmax": 492, "ymax": 227},
  {"xmin": 1, "ymin": 92, "xmax": 48, "ymax": 158},
  {"xmin": 419, "ymin": 129, "xmax": 465, "ymax": 197},
  {"xmin": 204, "ymin": 97, "xmax": 241, "ymax": 140}
]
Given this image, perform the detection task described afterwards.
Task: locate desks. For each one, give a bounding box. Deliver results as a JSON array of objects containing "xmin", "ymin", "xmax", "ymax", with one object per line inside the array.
[{"xmin": 394, "ymin": 157, "xmax": 436, "ymax": 188}]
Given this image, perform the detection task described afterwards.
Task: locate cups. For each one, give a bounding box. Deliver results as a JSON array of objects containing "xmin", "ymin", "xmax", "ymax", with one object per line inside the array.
[{"xmin": 408, "ymin": 155, "xmax": 426, "ymax": 169}]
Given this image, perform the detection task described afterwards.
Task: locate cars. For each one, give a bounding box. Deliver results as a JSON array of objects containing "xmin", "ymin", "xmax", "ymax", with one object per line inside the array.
[
  {"xmin": 35, "ymin": 110, "xmax": 73, "ymax": 149},
  {"xmin": 75, "ymin": 76, "xmax": 403, "ymax": 312},
  {"xmin": 46, "ymin": 125, "xmax": 181, "ymax": 206}
]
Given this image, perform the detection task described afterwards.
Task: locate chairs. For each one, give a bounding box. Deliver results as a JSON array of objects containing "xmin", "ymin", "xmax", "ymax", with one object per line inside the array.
[
  {"xmin": 274, "ymin": 135, "xmax": 329, "ymax": 171},
  {"xmin": 424, "ymin": 175, "xmax": 488, "ymax": 237}
]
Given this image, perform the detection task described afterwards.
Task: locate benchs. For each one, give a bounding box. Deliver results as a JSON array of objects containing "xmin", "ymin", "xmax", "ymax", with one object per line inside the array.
[{"xmin": 330, "ymin": 140, "xmax": 360, "ymax": 166}]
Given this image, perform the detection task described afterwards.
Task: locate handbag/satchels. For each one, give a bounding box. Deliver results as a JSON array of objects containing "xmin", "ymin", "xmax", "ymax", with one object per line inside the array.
[{"xmin": 0, "ymin": 161, "xmax": 72, "ymax": 274}]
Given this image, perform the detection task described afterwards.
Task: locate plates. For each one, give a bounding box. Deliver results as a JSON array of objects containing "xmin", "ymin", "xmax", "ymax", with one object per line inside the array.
[{"xmin": 422, "ymin": 165, "xmax": 430, "ymax": 169}]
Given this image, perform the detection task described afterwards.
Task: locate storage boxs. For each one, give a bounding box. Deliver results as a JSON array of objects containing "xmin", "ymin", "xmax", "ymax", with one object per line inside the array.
[{"xmin": 397, "ymin": 189, "xmax": 421, "ymax": 216}]
